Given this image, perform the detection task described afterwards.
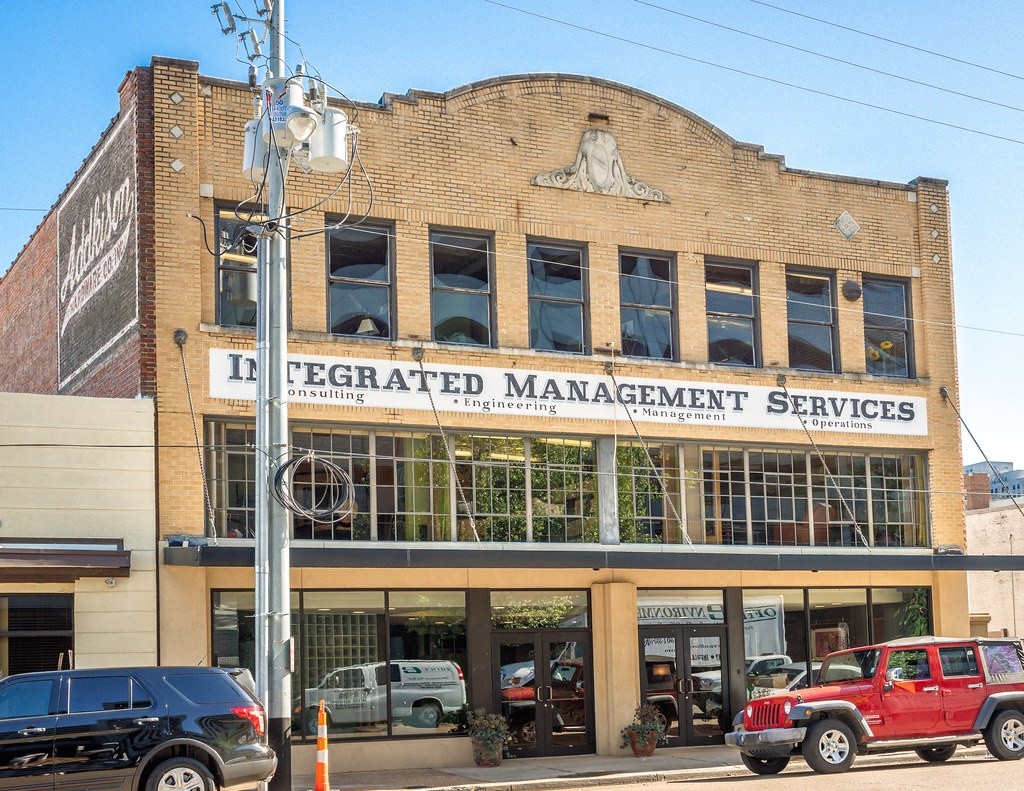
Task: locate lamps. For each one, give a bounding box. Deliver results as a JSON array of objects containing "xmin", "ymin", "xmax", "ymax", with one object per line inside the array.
[{"xmin": 356, "ymin": 315, "xmax": 379, "ymax": 337}]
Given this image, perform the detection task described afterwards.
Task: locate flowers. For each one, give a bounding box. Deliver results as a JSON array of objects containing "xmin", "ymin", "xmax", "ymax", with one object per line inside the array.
[{"xmin": 866, "ymin": 341, "xmax": 893, "ymax": 372}]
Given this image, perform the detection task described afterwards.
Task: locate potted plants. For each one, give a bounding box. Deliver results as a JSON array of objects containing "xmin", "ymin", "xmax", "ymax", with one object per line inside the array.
[
  {"xmin": 619, "ymin": 701, "xmax": 669, "ymax": 757},
  {"xmin": 463, "ymin": 708, "xmax": 519, "ymax": 767}
]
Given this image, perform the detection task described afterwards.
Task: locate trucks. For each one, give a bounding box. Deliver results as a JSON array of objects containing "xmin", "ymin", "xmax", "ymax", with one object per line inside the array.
[
  {"xmin": 550, "ymin": 595, "xmax": 787, "ymax": 713},
  {"xmin": 293, "ymin": 660, "xmax": 466, "ymax": 727}
]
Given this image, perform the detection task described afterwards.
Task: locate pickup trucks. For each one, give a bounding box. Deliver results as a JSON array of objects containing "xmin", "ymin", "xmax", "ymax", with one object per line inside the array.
[{"xmin": 691, "ymin": 655, "xmax": 793, "ymax": 713}]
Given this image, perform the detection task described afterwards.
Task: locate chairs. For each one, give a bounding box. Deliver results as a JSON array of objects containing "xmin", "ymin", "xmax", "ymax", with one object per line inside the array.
[{"xmin": 314, "ymin": 499, "xmax": 358, "ymax": 539}]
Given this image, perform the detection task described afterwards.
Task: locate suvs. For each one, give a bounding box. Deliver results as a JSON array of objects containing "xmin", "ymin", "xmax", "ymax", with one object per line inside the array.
[
  {"xmin": 501, "ymin": 656, "xmax": 679, "ymax": 743},
  {"xmin": 725, "ymin": 635, "xmax": 1024, "ymax": 774}
]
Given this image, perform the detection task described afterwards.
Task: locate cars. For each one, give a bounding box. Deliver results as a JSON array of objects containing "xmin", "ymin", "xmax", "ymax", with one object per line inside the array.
[
  {"xmin": 706, "ymin": 661, "xmax": 876, "ymax": 733},
  {"xmin": 0, "ymin": 666, "xmax": 278, "ymax": 791}
]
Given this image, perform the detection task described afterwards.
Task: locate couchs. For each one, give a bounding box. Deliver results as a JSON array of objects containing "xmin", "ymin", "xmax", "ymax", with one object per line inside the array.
[{"xmin": 775, "ymin": 505, "xmax": 837, "ymax": 545}]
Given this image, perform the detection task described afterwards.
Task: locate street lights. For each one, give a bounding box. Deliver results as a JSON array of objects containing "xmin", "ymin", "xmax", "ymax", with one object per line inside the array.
[{"xmin": 259, "ymin": 107, "xmax": 318, "ymax": 791}]
